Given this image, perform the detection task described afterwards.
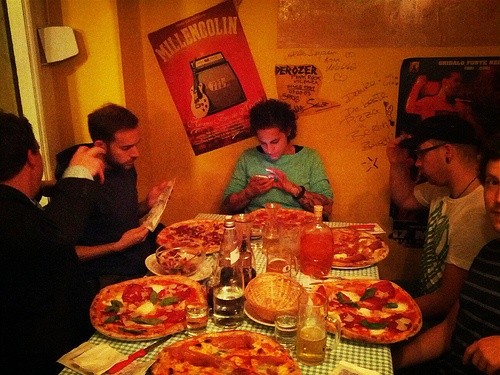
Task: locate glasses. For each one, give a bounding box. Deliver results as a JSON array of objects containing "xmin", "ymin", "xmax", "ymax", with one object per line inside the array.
[{"xmin": 409, "ymin": 141, "xmax": 448, "ymax": 160}]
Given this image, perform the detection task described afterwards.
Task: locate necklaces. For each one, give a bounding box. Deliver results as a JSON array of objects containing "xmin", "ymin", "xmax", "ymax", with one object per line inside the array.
[{"xmin": 456, "ymin": 177, "xmax": 478, "ymax": 198}]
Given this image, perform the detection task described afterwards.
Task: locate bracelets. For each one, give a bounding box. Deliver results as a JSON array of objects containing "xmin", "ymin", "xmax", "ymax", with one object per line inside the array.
[{"xmin": 291, "ymin": 185, "xmax": 305, "ymax": 200}]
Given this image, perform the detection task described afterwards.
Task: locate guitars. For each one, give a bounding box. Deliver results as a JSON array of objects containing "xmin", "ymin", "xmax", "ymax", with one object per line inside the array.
[{"xmin": 189, "ymin": 57, "xmax": 210, "ymax": 118}]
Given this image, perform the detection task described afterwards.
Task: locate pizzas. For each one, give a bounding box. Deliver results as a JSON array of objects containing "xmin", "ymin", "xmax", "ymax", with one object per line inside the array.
[
  {"xmin": 330, "ymin": 229, "xmax": 389, "ymax": 266},
  {"xmin": 312, "ymin": 280, "xmax": 421, "ymax": 343},
  {"xmin": 90, "ymin": 274, "xmax": 206, "ymax": 339},
  {"xmin": 156, "ymin": 218, "xmax": 238, "ymax": 255},
  {"xmin": 149, "ymin": 329, "xmax": 302, "ymax": 375},
  {"xmin": 242, "ymin": 207, "xmax": 319, "ymax": 237}
]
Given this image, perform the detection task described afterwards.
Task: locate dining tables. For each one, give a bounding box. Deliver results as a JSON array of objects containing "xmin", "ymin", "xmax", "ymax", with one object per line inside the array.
[{"xmin": 58, "ymin": 213, "xmax": 393, "ymax": 375}]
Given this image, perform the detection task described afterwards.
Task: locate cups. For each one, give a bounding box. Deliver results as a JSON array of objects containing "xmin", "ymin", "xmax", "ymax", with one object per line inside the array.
[
  {"xmin": 185, "ymin": 299, "xmax": 209, "ymax": 336},
  {"xmin": 274, "ymin": 291, "xmax": 341, "ymax": 366},
  {"xmin": 267, "ymin": 244, "xmax": 291, "ymax": 279}
]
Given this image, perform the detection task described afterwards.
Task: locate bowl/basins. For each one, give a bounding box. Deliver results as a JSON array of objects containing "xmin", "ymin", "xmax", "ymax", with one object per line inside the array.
[
  {"xmin": 155, "ymin": 241, "xmax": 205, "ymax": 277},
  {"xmin": 242, "ymin": 229, "xmax": 262, "ymax": 240}
]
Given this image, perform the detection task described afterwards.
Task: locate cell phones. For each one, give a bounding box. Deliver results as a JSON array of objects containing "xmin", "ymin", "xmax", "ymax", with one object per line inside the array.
[{"xmin": 254, "ymin": 174, "xmax": 267, "ymax": 181}]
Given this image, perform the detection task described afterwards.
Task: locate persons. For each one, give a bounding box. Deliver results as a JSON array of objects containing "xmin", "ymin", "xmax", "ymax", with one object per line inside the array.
[
  {"xmin": 384, "ymin": 113, "xmax": 500, "ymax": 342},
  {"xmin": 220, "ymin": 98, "xmax": 334, "ymax": 223},
  {"xmin": 0, "ymin": 107, "xmax": 105, "ymax": 375},
  {"xmin": 405, "ymin": 66, "xmax": 482, "ymax": 133},
  {"xmin": 391, "ymin": 135, "xmax": 500, "ymax": 375},
  {"xmin": 54, "ymin": 104, "xmax": 167, "ymax": 278}
]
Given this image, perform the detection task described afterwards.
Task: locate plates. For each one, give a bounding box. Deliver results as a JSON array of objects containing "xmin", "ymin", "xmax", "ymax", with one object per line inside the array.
[
  {"xmin": 89, "ymin": 275, "xmax": 207, "ymax": 342},
  {"xmin": 330, "ymin": 228, "xmax": 390, "ymax": 270},
  {"xmin": 243, "ymin": 297, "xmax": 313, "ymax": 326},
  {"xmin": 310, "ymin": 279, "xmax": 422, "ymax": 344},
  {"xmin": 156, "ymin": 218, "xmax": 239, "ymax": 256},
  {"xmin": 145, "ymin": 253, "xmax": 213, "ymax": 282},
  {"xmin": 140, "ymin": 329, "xmax": 303, "ymax": 375}
]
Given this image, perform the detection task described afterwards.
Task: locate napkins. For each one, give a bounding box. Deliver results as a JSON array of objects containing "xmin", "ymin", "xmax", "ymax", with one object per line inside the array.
[{"xmin": 72, "ymin": 342, "xmax": 126, "ymax": 375}]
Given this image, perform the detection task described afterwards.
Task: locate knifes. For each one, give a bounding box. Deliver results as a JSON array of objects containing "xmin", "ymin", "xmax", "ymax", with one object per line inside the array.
[{"xmin": 103, "ymin": 335, "xmax": 172, "ymax": 375}]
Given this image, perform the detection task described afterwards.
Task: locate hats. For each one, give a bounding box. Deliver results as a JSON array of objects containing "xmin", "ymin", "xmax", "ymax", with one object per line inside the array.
[{"xmin": 399, "ymin": 113, "xmax": 477, "ymax": 148}]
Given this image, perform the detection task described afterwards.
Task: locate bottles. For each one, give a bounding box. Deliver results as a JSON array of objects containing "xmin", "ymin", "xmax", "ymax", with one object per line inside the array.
[
  {"xmin": 298, "ymin": 205, "xmax": 333, "ymax": 279},
  {"xmin": 213, "ymin": 214, "xmax": 255, "ymax": 329},
  {"xmin": 261, "ymin": 203, "xmax": 282, "ymax": 249}
]
